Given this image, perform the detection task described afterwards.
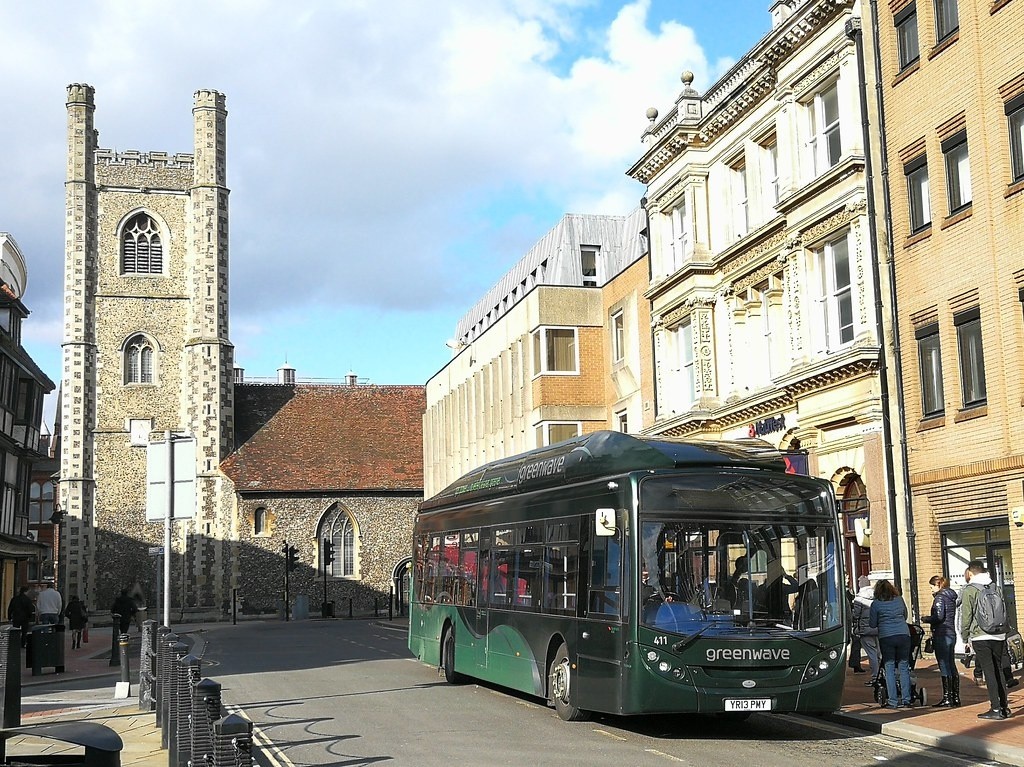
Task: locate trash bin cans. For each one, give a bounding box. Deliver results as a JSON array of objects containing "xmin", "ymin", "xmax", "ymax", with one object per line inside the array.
[
  {"xmin": 321, "ymin": 602, "xmax": 335, "ymax": 618},
  {"xmin": 27, "ymin": 624, "xmax": 67, "ymax": 675}
]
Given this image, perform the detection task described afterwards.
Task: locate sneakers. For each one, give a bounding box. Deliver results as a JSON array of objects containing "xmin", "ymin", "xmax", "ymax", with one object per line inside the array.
[
  {"xmin": 1006, "ymin": 678, "xmax": 1019, "ymax": 688},
  {"xmin": 977, "ymin": 708, "xmax": 1003, "ymax": 720},
  {"xmin": 1002, "ymin": 709, "xmax": 1012, "ymax": 718},
  {"xmin": 973, "ymin": 677, "xmax": 983, "ymax": 685}
]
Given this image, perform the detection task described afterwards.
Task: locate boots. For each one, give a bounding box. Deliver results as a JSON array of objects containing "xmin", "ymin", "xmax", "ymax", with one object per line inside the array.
[
  {"xmin": 931, "ymin": 675, "xmax": 953, "ymax": 707},
  {"xmin": 72, "ymin": 640, "xmax": 75, "ymax": 649},
  {"xmin": 77, "ymin": 642, "xmax": 81, "ymax": 649},
  {"xmin": 951, "ymin": 674, "xmax": 961, "ymax": 707}
]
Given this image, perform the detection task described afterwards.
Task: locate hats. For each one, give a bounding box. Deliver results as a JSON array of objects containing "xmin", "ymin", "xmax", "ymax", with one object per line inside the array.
[{"xmin": 858, "ymin": 575, "xmax": 869, "ymax": 588}]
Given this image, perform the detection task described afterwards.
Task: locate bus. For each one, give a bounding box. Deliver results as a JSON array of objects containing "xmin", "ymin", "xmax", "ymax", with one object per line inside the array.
[{"xmin": 407, "ymin": 430, "xmax": 874, "ymax": 722}]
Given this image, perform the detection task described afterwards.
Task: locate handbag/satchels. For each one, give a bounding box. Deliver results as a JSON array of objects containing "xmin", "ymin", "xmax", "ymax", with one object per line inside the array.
[
  {"xmin": 83, "ymin": 629, "xmax": 89, "ymax": 643},
  {"xmin": 924, "ymin": 638, "xmax": 934, "ymax": 653}
]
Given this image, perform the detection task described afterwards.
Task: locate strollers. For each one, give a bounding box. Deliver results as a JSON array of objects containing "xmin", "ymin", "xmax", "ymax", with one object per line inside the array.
[{"xmin": 873, "ymin": 622, "xmax": 927, "ymax": 708}]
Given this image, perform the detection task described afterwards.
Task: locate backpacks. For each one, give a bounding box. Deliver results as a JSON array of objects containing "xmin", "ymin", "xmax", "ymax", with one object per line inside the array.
[
  {"xmin": 1004, "ymin": 628, "xmax": 1024, "ymax": 669},
  {"xmin": 971, "ymin": 583, "xmax": 1008, "ymax": 634}
]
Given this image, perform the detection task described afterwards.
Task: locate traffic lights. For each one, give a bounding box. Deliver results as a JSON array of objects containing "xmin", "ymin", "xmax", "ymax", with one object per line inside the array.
[
  {"xmin": 325, "ymin": 540, "xmax": 336, "ymax": 565},
  {"xmin": 289, "ymin": 545, "xmax": 300, "ymax": 571}
]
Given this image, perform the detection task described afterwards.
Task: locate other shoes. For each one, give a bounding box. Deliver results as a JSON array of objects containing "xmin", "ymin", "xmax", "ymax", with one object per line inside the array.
[
  {"xmin": 961, "ymin": 659, "xmax": 970, "ymax": 669},
  {"xmin": 902, "ymin": 703, "xmax": 914, "ymax": 708},
  {"xmin": 854, "ymin": 667, "xmax": 867, "ymax": 674},
  {"xmin": 865, "ymin": 681, "xmax": 873, "ymax": 688},
  {"xmin": 885, "ymin": 702, "xmax": 893, "ymax": 709}
]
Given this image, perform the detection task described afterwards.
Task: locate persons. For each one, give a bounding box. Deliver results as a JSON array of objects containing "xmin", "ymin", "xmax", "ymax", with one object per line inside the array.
[
  {"xmin": 846, "ymin": 583, "xmax": 867, "ymax": 675},
  {"xmin": 65, "ymin": 595, "xmax": 88, "ymax": 649},
  {"xmin": 851, "ymin": 575, "xmax": 882, "ymax": 687},
  {"xmin": 641, "ymin": 571, "xmax": 672, "ymax": 610},
  {"xmin": 111, "ymin": 591, "xmax": 138, "ymax": 634},
  {"xmin": 8, "ymin": 586, "xmax": 33, "ymax": 648},
  {"xmin": 956, "ymin": 561, "xmax": 1019, "ymax": 720},
  {"xmin": 921, "ymin": 576, "xmax": 961, "ymax": 707},
  {"xmin": 36, "ymin": 582, "xmax": 62, "ymax": 633},
  {"xmin": 868, "ymin": 580, "xmax": 912, "ymax": 709}
]
